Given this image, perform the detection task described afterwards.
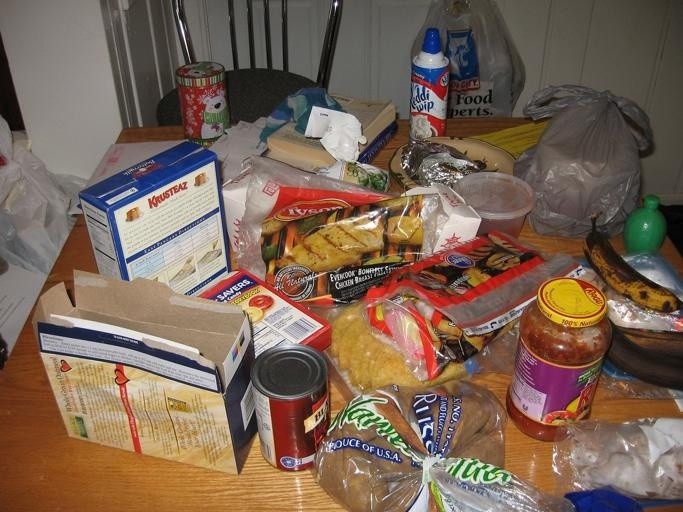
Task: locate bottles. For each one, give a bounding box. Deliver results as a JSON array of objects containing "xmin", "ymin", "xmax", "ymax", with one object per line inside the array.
[
  {"xmin": 409, "ymin": 25, "xmax": 451, "ymax": 146},
  {"xmin": 622, "ymin": 193, "xmax": 668, "ymax": 255}
]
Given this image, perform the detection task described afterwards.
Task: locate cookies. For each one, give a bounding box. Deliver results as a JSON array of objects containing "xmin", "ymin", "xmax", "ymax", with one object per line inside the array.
[{"xmin": 330, "ymin": 302, "xmax": 467, "ymax": 394}]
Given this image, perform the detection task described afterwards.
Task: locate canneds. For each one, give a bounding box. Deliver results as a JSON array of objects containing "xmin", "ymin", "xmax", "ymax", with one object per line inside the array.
[
  {"xmin": 175, "ymin": 61, "xmax": 231, "ymax": 148},
  {"xmin": 249, "ymin": 344, "xmax": 329, "ymax": 472},
  {"xmin": 506, "ymin": 277, "xmax": 613, "ymax": 442}
]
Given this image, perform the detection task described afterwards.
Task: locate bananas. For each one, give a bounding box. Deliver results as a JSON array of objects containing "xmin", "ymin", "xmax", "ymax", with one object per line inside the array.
[{"xmin": 583, "ymin": 232, "xmax": 683, "ymax": 312}]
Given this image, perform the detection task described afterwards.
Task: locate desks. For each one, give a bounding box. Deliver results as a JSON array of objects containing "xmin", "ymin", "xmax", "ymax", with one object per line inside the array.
[{"xmin": 0, "ymin": 114, "xmax": 683, "ymax": 510}]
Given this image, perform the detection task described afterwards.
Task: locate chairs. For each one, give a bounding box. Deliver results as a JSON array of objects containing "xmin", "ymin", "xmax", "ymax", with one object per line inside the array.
[{"xmin": 152, "ymin": 0, "xmax": 344, "ymax": 126}]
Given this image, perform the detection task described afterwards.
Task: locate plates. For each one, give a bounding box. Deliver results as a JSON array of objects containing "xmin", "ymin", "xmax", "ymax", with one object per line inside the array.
[{"xmin": 387, "ymin": 135, "xmax": 516, "ymax": 190}]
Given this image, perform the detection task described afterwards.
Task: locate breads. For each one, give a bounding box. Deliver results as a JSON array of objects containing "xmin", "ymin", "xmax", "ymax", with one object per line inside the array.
[{"xmin": 282, "ymin": 213, "xmax": 385, "ymax": 271}]
